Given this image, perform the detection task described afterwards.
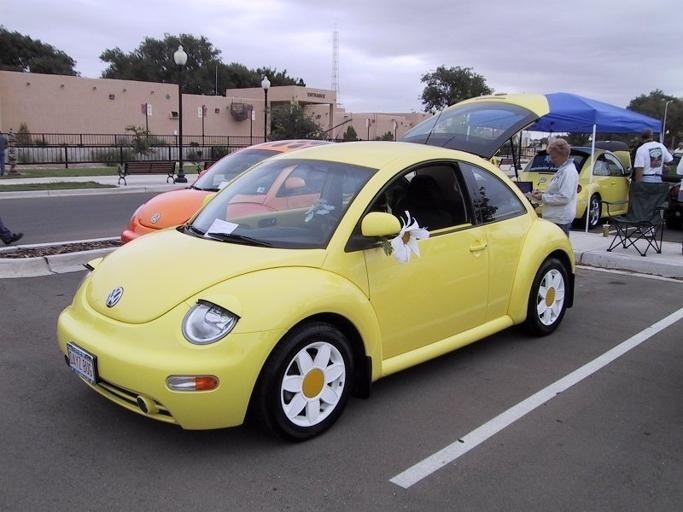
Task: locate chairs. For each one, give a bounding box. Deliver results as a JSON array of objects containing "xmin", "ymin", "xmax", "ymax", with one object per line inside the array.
[{"xmin": 598, "ymin": 181, "xmax": 669, "ymax": 257}]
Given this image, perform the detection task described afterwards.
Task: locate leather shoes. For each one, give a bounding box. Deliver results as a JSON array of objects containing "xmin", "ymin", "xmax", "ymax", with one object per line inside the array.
[{"xmin": 6, "ymin": 233, "xmax": 23, "ymax": 244}]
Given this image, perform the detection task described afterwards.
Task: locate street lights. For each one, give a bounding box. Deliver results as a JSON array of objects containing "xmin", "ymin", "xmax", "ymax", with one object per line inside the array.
[
  {"xmin": 174, "ymin": 44, "xmax": 188, "ymax": 183},
  {"xmin": 261, "ymin": 76, "xmax": 271, "ymax": 143},
  {"xmin": 661, "ymin": 98, "xmax": 674, "ymax": 142}
]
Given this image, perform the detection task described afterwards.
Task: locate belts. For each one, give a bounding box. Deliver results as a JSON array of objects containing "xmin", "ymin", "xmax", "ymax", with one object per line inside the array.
[{"xmin": 643, "ymin": 174, "xmax": 662, "ymax": 176}]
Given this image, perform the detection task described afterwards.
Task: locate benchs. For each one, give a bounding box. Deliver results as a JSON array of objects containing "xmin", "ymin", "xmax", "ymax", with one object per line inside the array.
[
  {"xmin": 118, "ymin": 160, "xmax": 176, "ymax": 186},
  {"xmin": 196, "ymin": 161, "xmax": 215, "ymax": 173}
]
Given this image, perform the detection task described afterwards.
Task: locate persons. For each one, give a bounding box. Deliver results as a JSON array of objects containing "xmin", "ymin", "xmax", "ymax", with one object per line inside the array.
[
  {"xmin": 674, "ymin": 142, "xmax": 683, "ymax": 153},
  {"xmin": 531, "ymin": 137, "xmax": 579, "ymax": 239},
  {"xmin": 633, "ymin": 128, "xmax": 673, "ymax": 238},
  {"xmin": 0, "ymin": 217, "xmax": 24, "ymax": 246},
  {"xmin": 0, "ymin": 130, "xmax": 9, "ymax": 177}
]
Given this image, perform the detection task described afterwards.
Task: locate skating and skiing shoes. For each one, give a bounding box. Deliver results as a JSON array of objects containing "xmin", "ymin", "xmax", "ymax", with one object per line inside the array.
[{"xmin": 643, "ymin": 226, "xmax": 656, "ymax": 237}]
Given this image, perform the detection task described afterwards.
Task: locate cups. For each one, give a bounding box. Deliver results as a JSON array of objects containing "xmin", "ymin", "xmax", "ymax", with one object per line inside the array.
[{"xmin": 603, "ymin": 224, "xmax": 610, "ymax": 237}]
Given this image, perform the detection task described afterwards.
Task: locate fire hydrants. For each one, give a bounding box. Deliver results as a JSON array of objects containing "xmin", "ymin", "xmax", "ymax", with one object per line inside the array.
[{"xmin": 8, "ymin": 149, "xmax": 17, "ymax": 172}]
